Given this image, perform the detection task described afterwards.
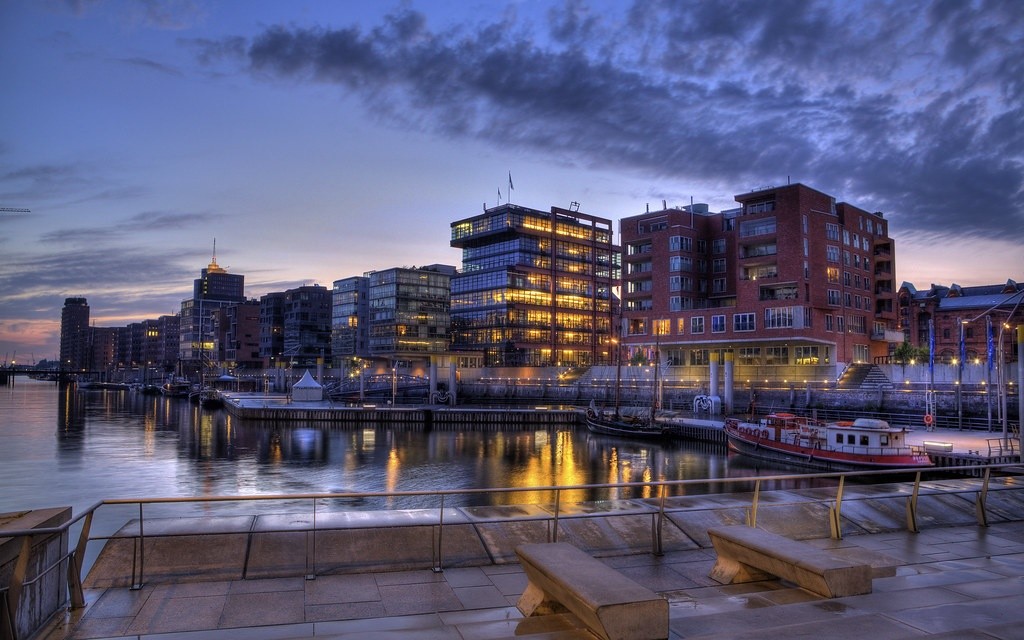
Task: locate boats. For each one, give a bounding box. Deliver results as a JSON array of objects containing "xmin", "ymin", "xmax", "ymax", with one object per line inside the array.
[
  {"xmin": 25, "ymin": 370, "xmax": 225, "ymax": 411},
  {"xmin": 721, "ymin": 411, "xmax": 936, "ymax": 486}
]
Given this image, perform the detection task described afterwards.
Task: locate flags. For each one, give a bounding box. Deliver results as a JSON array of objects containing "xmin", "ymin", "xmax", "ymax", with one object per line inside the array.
[
  {"xmin": 510, "ymin": 175, "xmax": 514, "ymax": 190},
  {"xmin": 498, "ymin": 191, "xmax": 501, "ymax": 198}
]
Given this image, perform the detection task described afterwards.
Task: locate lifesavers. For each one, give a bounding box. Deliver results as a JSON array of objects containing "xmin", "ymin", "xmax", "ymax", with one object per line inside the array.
[
  {"xmin": 837, "ymin": 422, "xmax": 852, "ymax": 427},
  {"xmin": 775, "ymin": 413, "xmax": 794, "ymax": 416},
  {"xmin": 924, "ymin": 414, "xmax": 932, "ymax": 424},
  {"xmin": 739, "ymin": 427, "xmax": 769, "ymax": 438},
  {"xmin": 656, "ymin": 401, "xmax": 660, "ymax": 407}
]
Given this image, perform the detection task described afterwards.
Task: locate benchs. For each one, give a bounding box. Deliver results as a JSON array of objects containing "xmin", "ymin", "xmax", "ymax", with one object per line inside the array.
[
  {"xmin": 508, "ymin": 542, "xmax": 670, "ymax": 640},
  {"xmin": 707, "ymin": 524, "xmax": 872, "ymax": 599}
]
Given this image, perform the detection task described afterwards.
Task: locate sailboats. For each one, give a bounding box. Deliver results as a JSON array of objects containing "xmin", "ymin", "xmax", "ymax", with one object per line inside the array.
[{"xmin": 583, "ymin": 322, "xmax": 682, "ymax": 444}]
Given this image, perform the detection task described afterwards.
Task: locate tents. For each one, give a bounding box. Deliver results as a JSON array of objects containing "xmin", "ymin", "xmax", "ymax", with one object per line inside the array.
[{"xmin": 292, "ymin": 369, "xmax": 322, "ymax": 401}]
